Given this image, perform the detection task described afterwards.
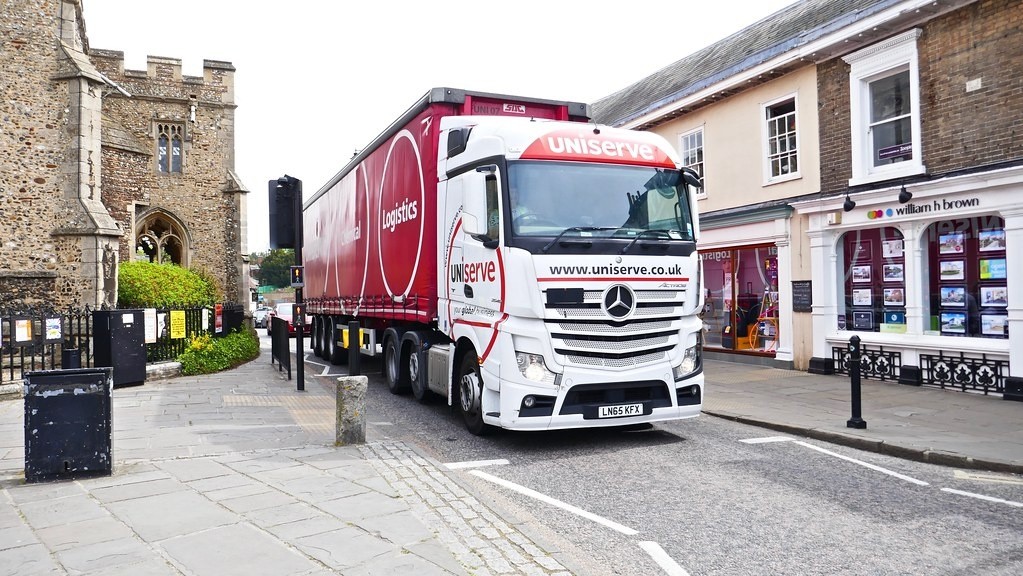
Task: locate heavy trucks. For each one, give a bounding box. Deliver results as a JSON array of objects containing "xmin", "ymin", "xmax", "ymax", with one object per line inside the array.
[{"xmin": 302, "ymin": 84, "xmax": 707, "ymax": 437}]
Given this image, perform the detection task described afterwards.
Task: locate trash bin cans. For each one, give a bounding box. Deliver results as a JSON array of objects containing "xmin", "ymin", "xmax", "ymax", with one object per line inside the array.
[{"xmin": 22, "ymin": 367, "xmax": 114, "ymax": 481}]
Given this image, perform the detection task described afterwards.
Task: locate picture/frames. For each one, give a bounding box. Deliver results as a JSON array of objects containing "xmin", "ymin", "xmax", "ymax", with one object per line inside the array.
[
  {"xmin": 936, "ymin": 227, "xmax": 1009, "ymax": 338},
  {"xmin": 882, "ymin": 285, "xmax": 906, "ymax": 309},
  {"xmin": 849, "ymin": 239, "xmax": 873, "ymax": 262},
  {"xmin": 880, "ymin": 237, "xmax": 904, "ymax": 262},
  {"xmin": 850, "ymin": 262, "xmax": 874, "ymax": 286},
  {"xmin": 881, "ymin": 309, "xmax": 906, "ymax": 324},
  {"xmin": 850, "ymin": 309, "xmax": 875, "ymax": 332},
  {"xmin": 880, "ymin": 261, "xmax": 905, "ymax": 285},
  {"xmin": 850, "ymin": 285, "xmax": 875, "ymax": 309}
]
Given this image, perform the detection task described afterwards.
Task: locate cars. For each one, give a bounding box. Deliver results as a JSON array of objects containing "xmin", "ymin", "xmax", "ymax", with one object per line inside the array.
[
  {"xmin": 267, "ymin": 302, "xmax": 311, "ymax": 338},
  {"xmin": 253, "ymin": 306, "xmax": 273, "ymax": 329}
]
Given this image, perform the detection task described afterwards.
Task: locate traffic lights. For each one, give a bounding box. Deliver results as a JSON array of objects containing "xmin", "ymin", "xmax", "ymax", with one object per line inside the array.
[{"xmin": 290, "ymin": 266, "xmax": 305, "ymax": 288}]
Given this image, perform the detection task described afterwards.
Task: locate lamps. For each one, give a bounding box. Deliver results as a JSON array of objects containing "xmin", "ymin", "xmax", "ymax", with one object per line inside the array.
[
  {"xmin": 844, "ymin": 184, "xmax": 874, "ymax": 212},
  {"xmin": 899, "ymin": 174, "xmax": 931, "ymax": 205}
]
